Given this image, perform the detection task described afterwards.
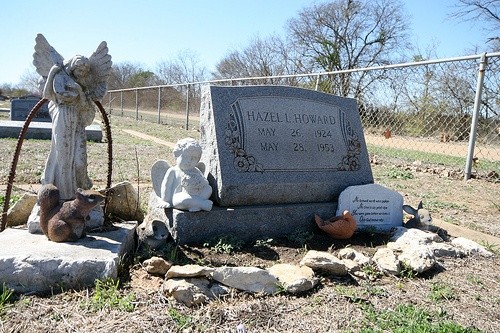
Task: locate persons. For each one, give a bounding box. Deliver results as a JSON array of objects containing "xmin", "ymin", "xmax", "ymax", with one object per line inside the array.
[
  {"xmin": 31, "ymin": 32, "xmax": 112, "ymax": 200},
  {"xmin": 150, "ymin": 137, "xmax": 214, "ymax": 213}
]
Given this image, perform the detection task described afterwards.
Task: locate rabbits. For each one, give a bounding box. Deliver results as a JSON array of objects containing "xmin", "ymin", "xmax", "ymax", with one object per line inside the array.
[{"xmin": 402, "ymin": 200, "xmax": 434, "ymax": 232}]
